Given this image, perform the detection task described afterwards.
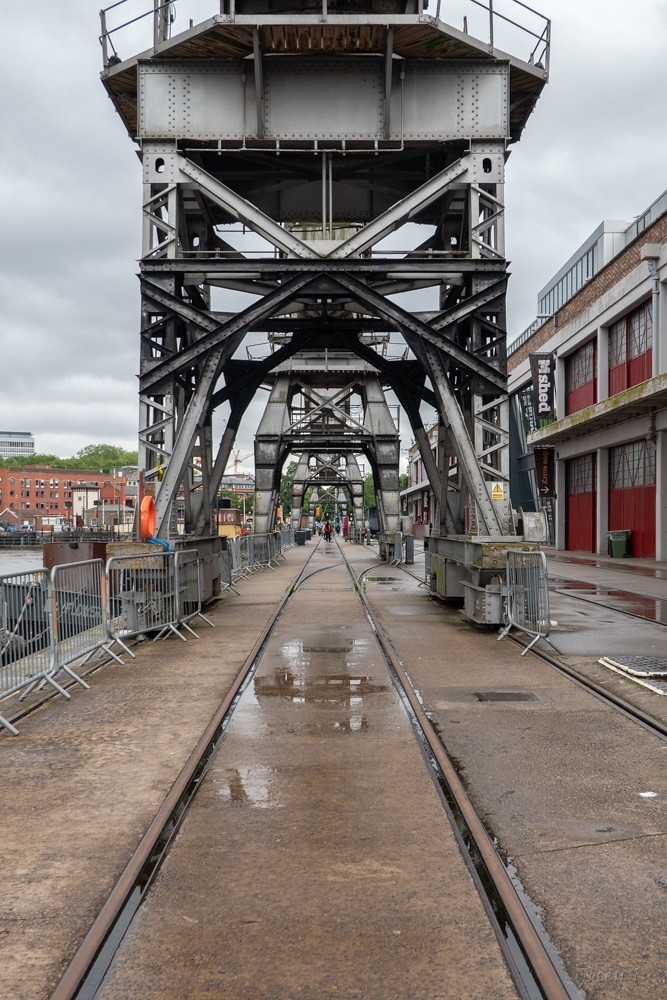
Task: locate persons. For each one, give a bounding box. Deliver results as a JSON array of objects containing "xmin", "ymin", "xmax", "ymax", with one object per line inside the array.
[
  {"xmin": 321, "ymin": 521, "xmax": 341, "ymax": 542},
  {"xmin": 0, "ymin": 523, "xmax": 16, "ymax": 532}
]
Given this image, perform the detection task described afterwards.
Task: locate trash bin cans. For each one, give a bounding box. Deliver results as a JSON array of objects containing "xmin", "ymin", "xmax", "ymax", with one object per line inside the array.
[
  {"xmin": 607, "ymin": 530, "xmax": 631, "ymax": 558},
  {"xmin": 296, "ymin": 531, "xmax": 307, "ymax": 546},
  {"xmin": 306, "ymin": 530, "xmax": 312, "ymax": 540}
]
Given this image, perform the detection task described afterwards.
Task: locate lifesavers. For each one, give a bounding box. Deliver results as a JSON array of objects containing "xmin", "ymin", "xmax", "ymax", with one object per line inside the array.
[{"xmin": 139, "ymin": 495, "xmax": 156, "ymax": 539}]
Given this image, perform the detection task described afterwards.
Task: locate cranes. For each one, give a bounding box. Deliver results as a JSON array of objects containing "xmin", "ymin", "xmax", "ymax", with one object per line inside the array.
[{"xmin": 227, "ymin": 449, "xmax": 254, "ymax": 475}]
